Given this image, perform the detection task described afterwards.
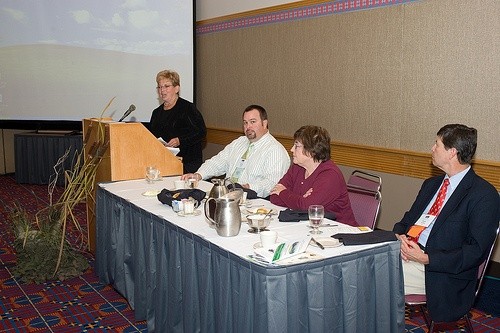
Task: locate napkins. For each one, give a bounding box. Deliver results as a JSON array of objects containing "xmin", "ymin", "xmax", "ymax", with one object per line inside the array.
[{"xmin": 309, "ymin": 236, "xmax": 340, "ymax": 246}]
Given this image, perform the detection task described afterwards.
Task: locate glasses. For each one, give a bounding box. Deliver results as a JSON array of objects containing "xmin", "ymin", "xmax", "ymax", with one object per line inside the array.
[
  {"xmin": 156, "ymin": 85, "xmax": 173, "ymax": 90},
  {"xmin": 293, "ymin": 144, "xmax": 304, "ymax": 149}
]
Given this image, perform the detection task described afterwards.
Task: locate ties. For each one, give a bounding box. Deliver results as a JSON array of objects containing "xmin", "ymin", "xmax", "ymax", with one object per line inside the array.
[
  {"xmin": 407, "ymin": 178, "xmax": 450, "ymax": 248},
  {"xmin": 231, "ymin": 143, "xmax": 253, "ymax": 183}
]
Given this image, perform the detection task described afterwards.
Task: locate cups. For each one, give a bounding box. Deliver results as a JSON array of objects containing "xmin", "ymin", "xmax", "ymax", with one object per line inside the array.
[
  {"xmin": 145, "ymin": 166, "xmax": 159, "ymax": 183},
  {"xmin": 174, "ymin": 180, "xmax": 185, "ymax": 189},
  {"xmin": 182, "ymin": 199, "xmax": 198, "ymax": 215},
  {"xmin": 185, "ymin": 174, "xmax": 198, "ymax": 189},
  {"xmin": 260, "ymin": 231, "xmax": 277, "ymax": 248}
]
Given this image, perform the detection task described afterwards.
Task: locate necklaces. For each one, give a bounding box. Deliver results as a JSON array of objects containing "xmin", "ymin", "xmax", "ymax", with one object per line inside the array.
[{"xmin": 164, "ymin": 98, "xmax": 177, "ymax": 106}]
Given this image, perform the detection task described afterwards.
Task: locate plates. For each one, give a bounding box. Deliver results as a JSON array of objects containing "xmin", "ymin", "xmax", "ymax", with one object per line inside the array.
[
  {"xmin": 310, "ymin": 237, "xmax": 341, "ymax": 247},
  {"xmin": 178, "ymin": 209, "xmax": 201, "ymax": 217},
  {"xmin": 253, "ymin": 240, "xmax": 280, "ymax": 250}
]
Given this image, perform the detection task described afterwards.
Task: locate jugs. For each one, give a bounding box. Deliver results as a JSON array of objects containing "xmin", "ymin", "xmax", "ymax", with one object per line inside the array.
[
  {"xmin": 208, "ymin": 178, "xmax": 236, "ymax": 224},
  {"xmin": 204, "ymin": 196, "xmax": 242, "ymax": 237}
]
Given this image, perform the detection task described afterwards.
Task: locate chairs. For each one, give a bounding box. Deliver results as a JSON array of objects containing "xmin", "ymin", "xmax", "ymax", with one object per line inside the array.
[{"xmin": 346, "ymin": 170, "xmax": 500, "ymax": 333}]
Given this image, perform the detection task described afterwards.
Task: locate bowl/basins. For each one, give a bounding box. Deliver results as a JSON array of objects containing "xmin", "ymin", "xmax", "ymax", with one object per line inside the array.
[{"xmin": 246, "ymin": 213, "xmax": 271, "ymax": 232}]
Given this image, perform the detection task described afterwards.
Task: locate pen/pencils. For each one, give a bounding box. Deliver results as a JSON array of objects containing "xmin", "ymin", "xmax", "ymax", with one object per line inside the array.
[{"xmin": 307, "ymin": 234, "xmax": 324, "ymax": 250}]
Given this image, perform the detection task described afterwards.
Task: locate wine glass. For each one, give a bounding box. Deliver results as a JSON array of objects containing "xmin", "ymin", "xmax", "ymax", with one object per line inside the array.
[{"xmin": 308, "ymin": 205, "xmax": 323, "ymax": 235}]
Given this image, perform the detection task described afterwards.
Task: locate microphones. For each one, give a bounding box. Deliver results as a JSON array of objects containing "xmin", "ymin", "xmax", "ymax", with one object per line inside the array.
[{"xmin": 119, "ymin": 105, "xmax": 136, "ymax": 122}]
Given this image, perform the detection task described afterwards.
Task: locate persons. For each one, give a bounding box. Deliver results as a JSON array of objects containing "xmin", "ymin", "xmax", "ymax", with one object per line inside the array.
[
  {"xmin": 392, "ymin": 123, "xmax": 500, "ymax": 321},
  {"xmin": 181, "ymin": 105, "xmax": 292, "ymax": 198},
  {"xmin": 270, "ymin": 125, "xmax": 359, "ymax": 227},
  {"xmin": 148, "ymin": 70, "xmax": 205, "ymax": 174}
]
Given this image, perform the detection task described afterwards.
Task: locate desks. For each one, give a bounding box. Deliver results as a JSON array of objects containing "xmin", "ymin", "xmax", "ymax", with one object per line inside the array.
[
  {"xmin": 88, "ymin": 172, "xmax": 405, "ymax": 333},
  {"xmin": 13, "ymin": 130, "xmax": 84, "ymax": 187}
]
